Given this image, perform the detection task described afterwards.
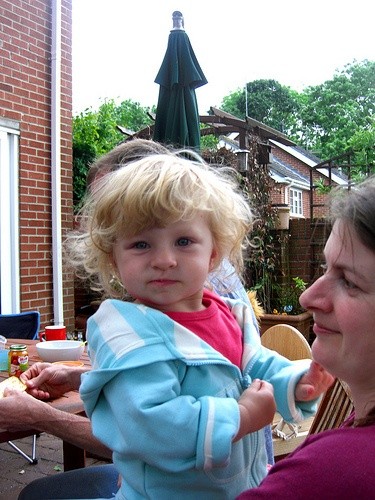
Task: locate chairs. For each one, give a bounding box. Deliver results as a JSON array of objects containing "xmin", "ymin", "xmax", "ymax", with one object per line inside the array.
[
  {"xmin": 260, "ymin": 324, "xmax": 313, "ymax": 361},
  {"xmin": 0, "ymin": 310, "xmax": 40, "ymax": 465},
  {"xmin": 272, "ymin": 379, "xmax": 355, "ymax": 460}
]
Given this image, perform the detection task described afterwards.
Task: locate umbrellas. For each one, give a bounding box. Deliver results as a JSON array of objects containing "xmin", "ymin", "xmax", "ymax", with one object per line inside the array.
[{"xmin": 153, "ymin": 11, "xmax": 208, "ymax": 163}]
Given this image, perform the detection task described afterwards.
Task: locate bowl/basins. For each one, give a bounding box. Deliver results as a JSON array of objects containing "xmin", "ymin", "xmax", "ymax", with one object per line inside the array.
[{"xmin": 36, "ymin": 340, "xmax": 85, "ymax": 363}]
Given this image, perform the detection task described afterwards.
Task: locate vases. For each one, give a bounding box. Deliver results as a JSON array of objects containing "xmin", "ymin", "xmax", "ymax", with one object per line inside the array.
[{"xmin": 259, "ymin": 311, "xmax": 313, "ymax": 344}]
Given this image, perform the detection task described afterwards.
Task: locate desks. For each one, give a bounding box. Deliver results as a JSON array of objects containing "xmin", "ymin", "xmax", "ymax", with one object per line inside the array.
[{"xmin": 1, "ymin": 338, "xmax": 92, "ymax": 472}]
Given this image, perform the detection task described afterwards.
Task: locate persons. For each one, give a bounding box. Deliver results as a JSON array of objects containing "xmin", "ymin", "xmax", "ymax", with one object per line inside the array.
[
  {"xmin": 235, "ymin": 176, "xmax": 375, "ymax": 500},
  {"xmin": 69, "ymin": 153, "xmax": 335, "ymax": 500},
  {"xmin": 0, "ymin": 140, "xmax": 259, "ymax": 500}
]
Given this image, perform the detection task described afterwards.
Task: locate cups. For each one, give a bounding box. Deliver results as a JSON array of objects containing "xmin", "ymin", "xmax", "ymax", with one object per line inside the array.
[
  {"xmin": 0, "ymin": 350, "xmax": 10, "ymax": 370},
  {"xmin": 39, "ymin": 325, "xmax": 66, "ymax": 341}
]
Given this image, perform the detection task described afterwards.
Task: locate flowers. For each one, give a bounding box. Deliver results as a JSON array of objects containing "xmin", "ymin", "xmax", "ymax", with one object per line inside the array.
[{"xmin": 250, "ymin": 256, "xmax": 308, "ymax": 316}]
